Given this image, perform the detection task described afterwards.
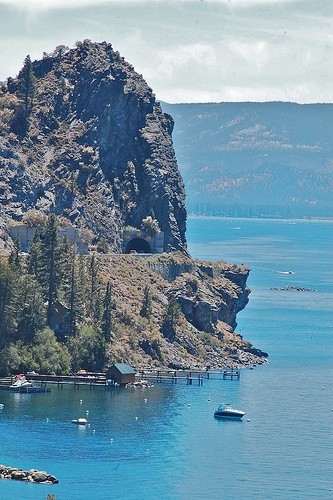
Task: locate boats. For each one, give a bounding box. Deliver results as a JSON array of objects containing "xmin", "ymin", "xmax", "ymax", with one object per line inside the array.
[
  {"xmin": 281, "ymin": 271, "xmax": 295, "ymax": 275},
  {"xmin": 214, "ymin": 403, "xmax": 246, "ymax": 421}
]
